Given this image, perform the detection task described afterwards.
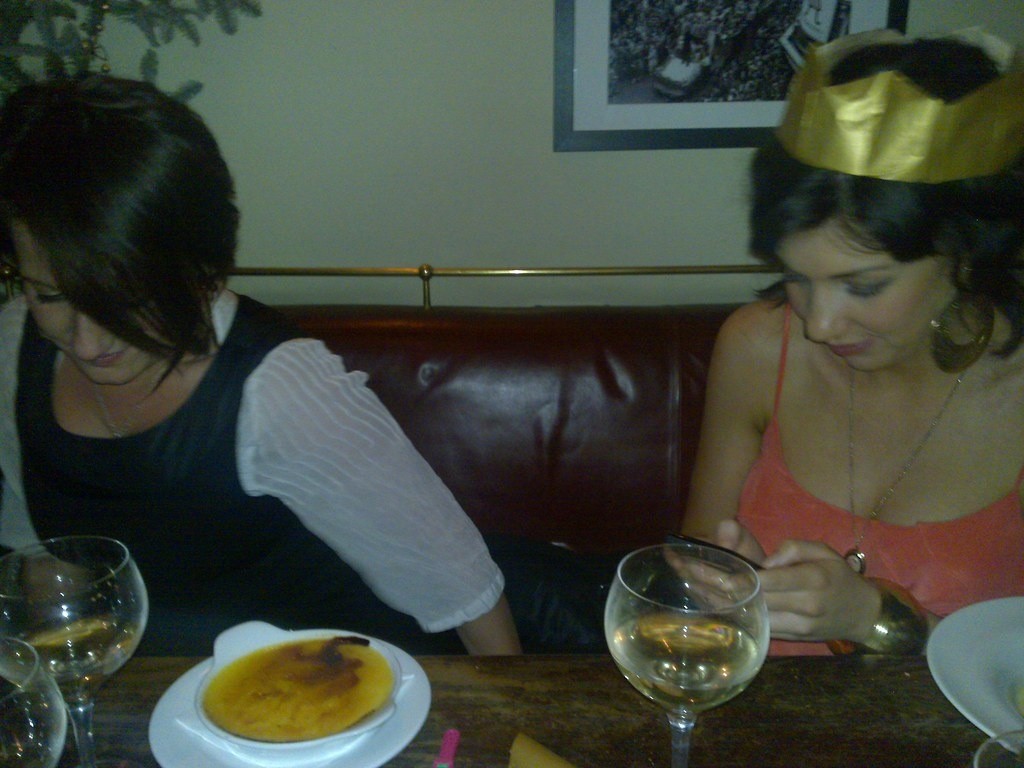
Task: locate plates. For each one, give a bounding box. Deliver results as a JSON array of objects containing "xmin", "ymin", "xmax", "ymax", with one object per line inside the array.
[
  {"xmin": 926, "ymin": 596, "xmax": 1024, "ymax": 753},
  {"xmin": 147, "ymin": 621, "xmax": 431, "ymax": 768}
]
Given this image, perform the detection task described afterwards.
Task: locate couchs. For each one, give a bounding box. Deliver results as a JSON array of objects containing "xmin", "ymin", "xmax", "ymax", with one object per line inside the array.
[{"xmin": 267, "ymin": 303, "xmax": 748, "ymax": 557}]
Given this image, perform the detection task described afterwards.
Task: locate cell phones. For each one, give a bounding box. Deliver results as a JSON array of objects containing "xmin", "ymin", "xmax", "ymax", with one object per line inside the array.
[{"xmin": 667, "ymin": 534, "xmax": 764, "ymax": 576}]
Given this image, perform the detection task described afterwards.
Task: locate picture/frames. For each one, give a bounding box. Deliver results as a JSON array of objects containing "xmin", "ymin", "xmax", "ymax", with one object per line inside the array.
[{"xmin": 550, "ymin": 1, "xmax": 911, "ymax": 153}]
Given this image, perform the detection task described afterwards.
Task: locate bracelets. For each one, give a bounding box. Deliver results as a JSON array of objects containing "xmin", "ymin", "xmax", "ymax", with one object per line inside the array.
[{"xmin": 863, "ymin": 579, "xmax": 928, "ymax": 657}]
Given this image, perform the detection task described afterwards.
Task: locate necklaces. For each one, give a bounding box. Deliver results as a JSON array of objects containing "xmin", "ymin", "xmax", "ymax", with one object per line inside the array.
[
  {"xmin": 89, "ymin": 380, "xmax": 148, "ymax": 437},
  {"xmin": 844, "ymin": 362, "xmax": 964, "ymax": 577}
]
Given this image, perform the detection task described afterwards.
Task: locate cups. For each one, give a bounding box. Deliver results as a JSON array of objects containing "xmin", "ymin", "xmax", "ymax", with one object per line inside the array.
[
  {"xmin": 0, "ymin": 636, "xmax": 67, "ymax": 768},
  {"xmin": 973, "ymin": 730, "xmax": 1024, "ymax": 768}
]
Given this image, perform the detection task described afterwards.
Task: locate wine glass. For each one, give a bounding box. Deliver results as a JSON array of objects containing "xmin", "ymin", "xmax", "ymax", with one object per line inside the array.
[
  {"xmin": 603, "ymin": 543, "xmax": 770, "ymax": 768},
  {"xmin": 0, "ymin": 535, "xmax": 149, "ymax": 768}
]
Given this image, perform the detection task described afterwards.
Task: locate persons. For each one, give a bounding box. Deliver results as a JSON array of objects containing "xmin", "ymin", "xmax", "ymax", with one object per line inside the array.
[
  {"xmin": 0, "ymin": 71, "xmax": 519, "ymax": 661},
  {"xmin": 662, "ymin": 23, "xmax": 1024, "ymax": 657}
]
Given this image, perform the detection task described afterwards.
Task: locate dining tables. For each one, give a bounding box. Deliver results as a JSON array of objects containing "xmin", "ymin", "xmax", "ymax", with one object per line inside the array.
[{"xmin": 0, "ymin": 651, "xmax": 1024, "ymax": 768}]
